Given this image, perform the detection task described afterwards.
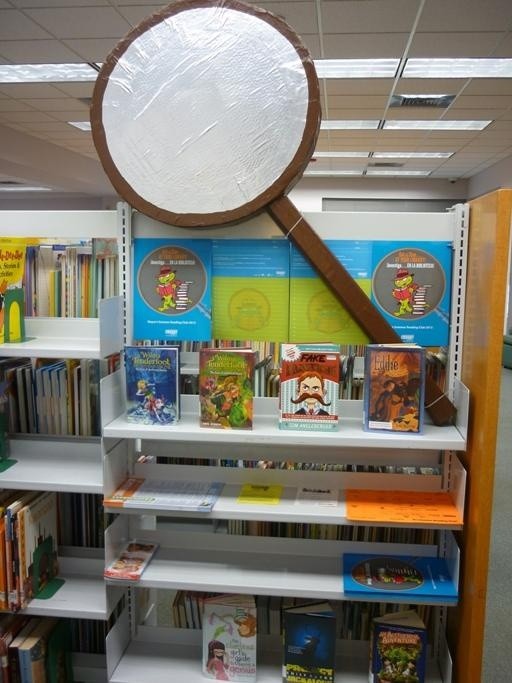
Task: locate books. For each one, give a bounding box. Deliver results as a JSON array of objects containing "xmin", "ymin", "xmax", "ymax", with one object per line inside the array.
[
  {"xmin": 120, "ymin": 338, "xmax": 449, "ymax": 435},
  {"xmin": 137, "ymin": 456, "xmax": 441, "ymax": 546},
  {"xmin": 342, "ymin": 554, "xmax": 458, "ymax": 602},
  {"xmin": 172, "ymin": 590, "xmax": 434, "ymax": 682},
  {"xmin": 0, "ymin": 232, "xmax": 113, "ymax": 437},
  {"xmin": 0, "ymin": 489, "xmax": 110, "ymax": 681}
]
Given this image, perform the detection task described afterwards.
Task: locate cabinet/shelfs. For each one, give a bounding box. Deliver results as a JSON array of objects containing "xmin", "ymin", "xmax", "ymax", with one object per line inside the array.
[{"xmin": 0, "ymin": 316, "xmax": 502, "ymax": 683}]
